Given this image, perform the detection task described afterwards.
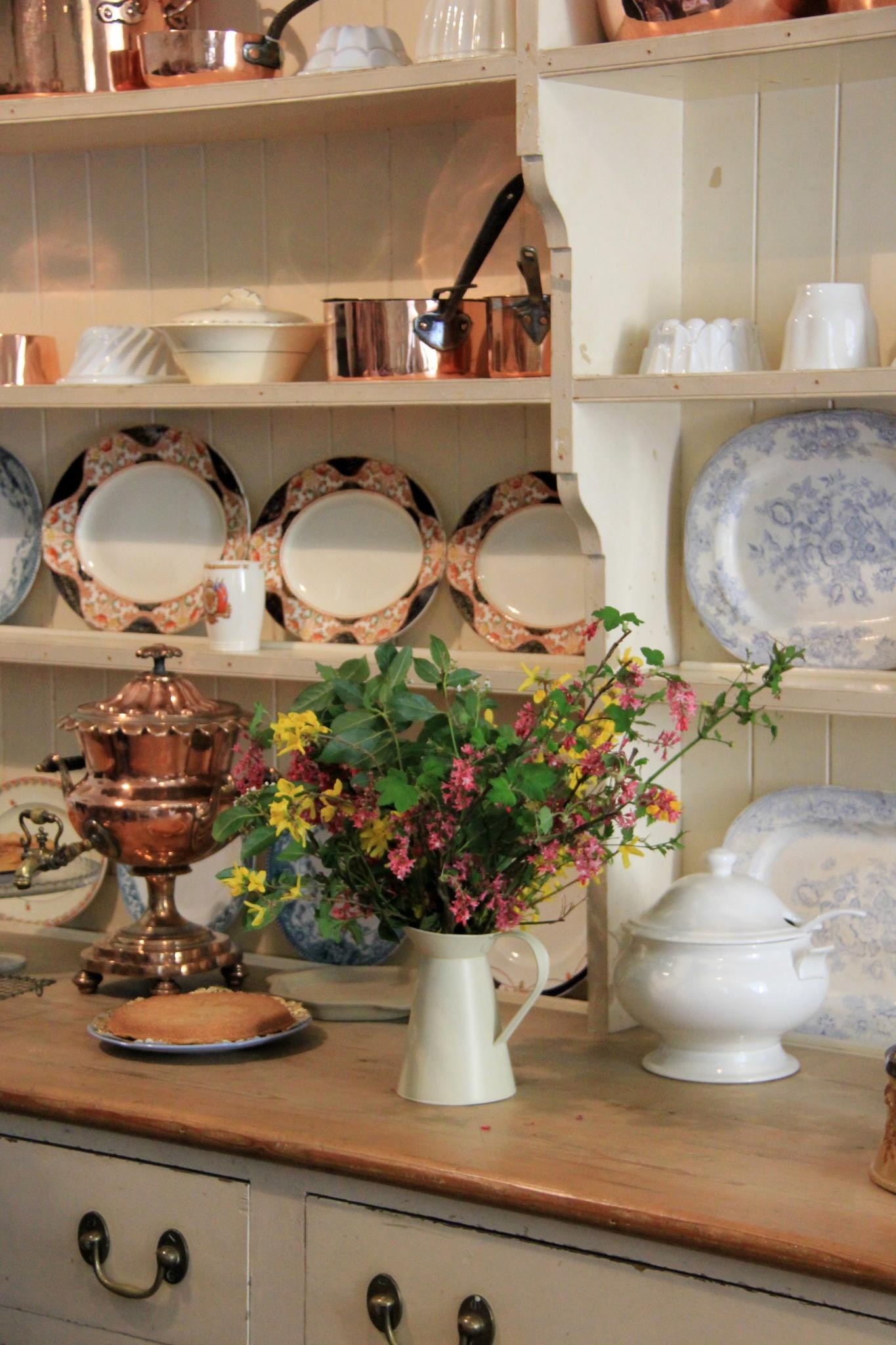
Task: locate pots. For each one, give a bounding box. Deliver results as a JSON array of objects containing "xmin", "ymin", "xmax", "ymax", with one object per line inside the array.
[
  {"xmin": 321, "ymin": 173, "xmax": 524, "ymax": 380},
  {"xmin": 482, "ymin": 246, "xmax": 551, "ymax": 379},
  {"xmin": 1, "ymin": 0, "xmax": 202, "ymax": 95},
  {"xmin": 136, "ymin": 0, "xmax": 320, "ymax": 87}
]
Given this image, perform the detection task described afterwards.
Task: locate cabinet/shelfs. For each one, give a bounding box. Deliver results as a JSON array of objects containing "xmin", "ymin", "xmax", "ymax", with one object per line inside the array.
[
  {"xmin": 1, "ymin": 0, "xmax": 896, "ymax": 1059},
  {"xmin": 1, "ymin": 982, "xmax": 895, "ymax": 1345}
]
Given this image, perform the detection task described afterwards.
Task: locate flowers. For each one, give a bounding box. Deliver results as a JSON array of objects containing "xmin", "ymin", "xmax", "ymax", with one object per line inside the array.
[{"xmin": 189, "ymin": 608, "xmax": 800, "ymax": 932}]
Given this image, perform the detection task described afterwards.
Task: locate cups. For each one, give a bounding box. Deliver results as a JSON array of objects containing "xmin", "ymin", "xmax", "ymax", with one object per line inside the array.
[
  {"xmin": 779, "ymin": 282, "xmax": 882, "ymax": 370},
  {"xmin": 201, "ymin": 560, "xmax": 266, "ymax": 652}
]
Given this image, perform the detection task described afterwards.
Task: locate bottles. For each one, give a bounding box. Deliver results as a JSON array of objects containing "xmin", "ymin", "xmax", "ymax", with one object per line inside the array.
[{"xmin": 611, "ymin": 843, "xmax": 869, "ymax": 1085}]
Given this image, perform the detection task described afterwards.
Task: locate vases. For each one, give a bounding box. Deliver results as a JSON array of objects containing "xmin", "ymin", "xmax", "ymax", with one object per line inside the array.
[{"xmin": 399, "ymin": 929, "xmax": 543, "ymax": 1102}]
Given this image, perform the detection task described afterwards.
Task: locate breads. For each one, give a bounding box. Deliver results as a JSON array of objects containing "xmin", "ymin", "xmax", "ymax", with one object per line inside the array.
[{"xmin": 103, "ymin": 990, "xmax": 292, "ymax": 1045}]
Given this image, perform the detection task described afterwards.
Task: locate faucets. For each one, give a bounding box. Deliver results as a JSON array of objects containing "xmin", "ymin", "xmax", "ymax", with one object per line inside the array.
[{"xmin": 12, "ymin": 805, "xmax": 120, "ymax": 890}]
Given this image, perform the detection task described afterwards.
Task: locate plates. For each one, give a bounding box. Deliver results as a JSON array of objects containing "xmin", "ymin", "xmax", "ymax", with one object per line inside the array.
[
  {"xmin": 0, "ymin": 776, "xmax": 590, "ymax": 1054},
  {"xmin": 446, "ymin": 470, "xmax": 586, "ymax": 655},
  {"xmin": 718, "ymin": 785, "xmax": 895, "ymax": 1050},
  {"xmin": 683, "ymin": 408, "xmax": 896, "ymax": 673},
  {"xmin": 0, "ymin": 445, "xmax": 44, "ymax": 626},
  {"xmin": 41, "ymin": 424, "xmax": 251, "ymax": 635},
  {"xmin": 250, "ymin": 456, "xmax": 447, "ymax": 645}
]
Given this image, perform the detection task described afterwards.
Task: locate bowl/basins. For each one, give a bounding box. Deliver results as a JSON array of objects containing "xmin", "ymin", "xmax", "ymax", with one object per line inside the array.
[
  {"xmin": 143, "ymin": 287, "xmax": 327, "ymax": 383},
  {"xmin": 637, "ymin": 318, "xmax": 772, "ymax": 376},
  {"xmin": 296, "ymin": 25, "xmax": 412, "ymax": 77},
  {"xmin": 0, "ymin": 333, "xmax": 65, "ymax": 387},
  {"xmin": 416, "ymin": 0, "xmax": 517, "ymax": 66},
  {"xmin": 55, "ymin": 324, "xmax": 190, "ymax": 385}
]
